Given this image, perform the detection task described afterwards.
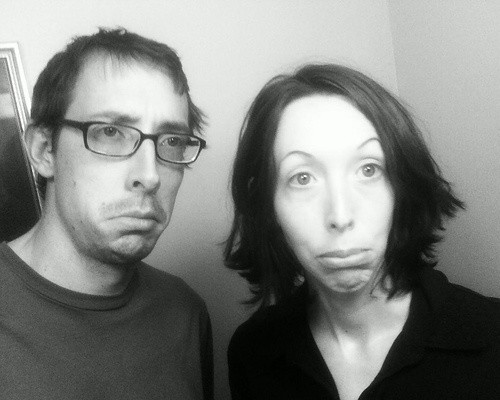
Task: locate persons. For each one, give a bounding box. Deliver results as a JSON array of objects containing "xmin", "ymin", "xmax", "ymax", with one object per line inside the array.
[
  {"xmin": 227, "ymin": 64, "xmax": 500, "ymax": 400},
  {"xmin": 0, "ymin": 28, "xmax": 212, "ymax": 400}
]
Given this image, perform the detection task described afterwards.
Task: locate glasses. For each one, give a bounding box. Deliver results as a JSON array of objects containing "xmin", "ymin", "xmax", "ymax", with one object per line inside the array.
[{"xmin": 44, "ymin": 118, "xmax": 206, "ymax": 165}]
